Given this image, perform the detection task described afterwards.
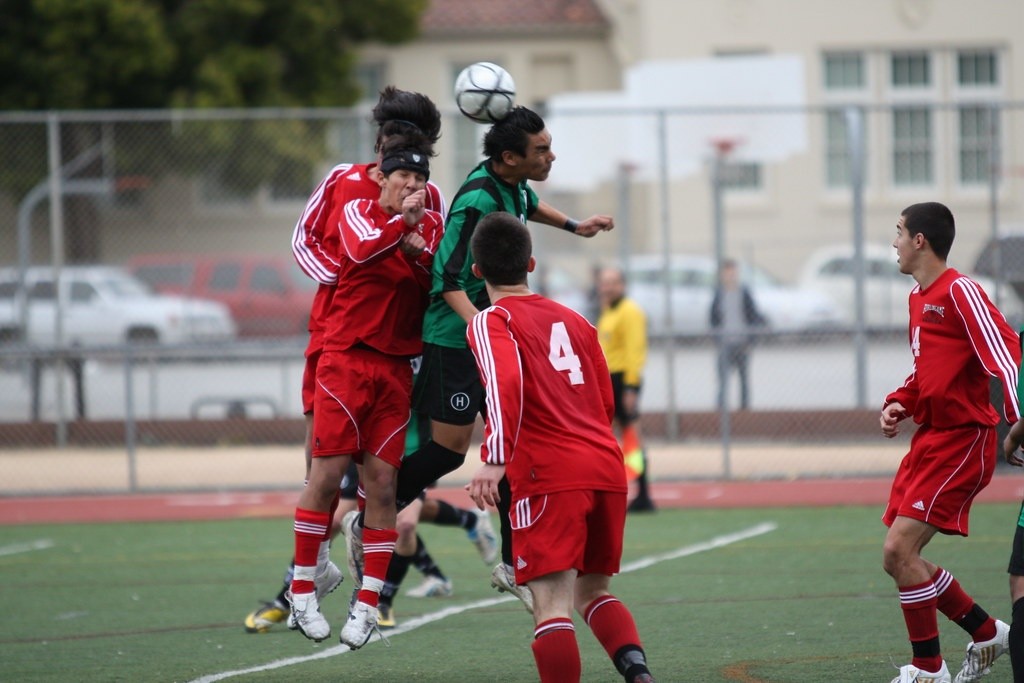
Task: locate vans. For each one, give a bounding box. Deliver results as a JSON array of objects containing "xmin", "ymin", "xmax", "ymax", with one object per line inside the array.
[{"xmin": 123, "ymin": 248, "xmax": 321, "ymax": 337}]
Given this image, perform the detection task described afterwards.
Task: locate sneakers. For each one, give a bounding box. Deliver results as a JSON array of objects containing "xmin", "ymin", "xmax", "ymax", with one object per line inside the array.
[
  {"xmin": 889, "ymin": 653, "xmax": 951, "ymax": 683},
  {"xmin": 285, "ymin": 559, "xmax": 344, "ymax": 629},
  {"xmin": 468, "ymin": 506, "xmax": 498, "ymax": 566},
  {"xmin": 284, "ymin": 581, "xmax": 332, "ymax": 644},
  {"xmin": 244, "ymin": 598, "xmax": 291, "ymax": 634},
  {"xmin": 376, "ymin": 601, "xmax": 397, "ymax": 629},
  {"xmin": 956, "ymin": 618, "xmax": 1011, "ymax": 683},
  {"xmin": 407, "ymin": 575, "xmax": 453, "ymax": 599},
  {"xmin": 491, "ymin": 561, "xmax": 535, "ymax": 613},
  {"xmin": 340, "ymin": 599, "xmax": 379, "ymax": 650}
]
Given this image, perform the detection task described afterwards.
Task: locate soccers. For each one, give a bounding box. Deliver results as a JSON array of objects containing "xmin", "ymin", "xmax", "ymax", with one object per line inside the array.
[{"xmin": 454, "ymin": 60, "xmax": 518, "ymax": 123}]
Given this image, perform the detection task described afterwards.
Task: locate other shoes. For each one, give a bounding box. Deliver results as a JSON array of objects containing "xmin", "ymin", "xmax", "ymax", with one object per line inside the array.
[{"xmin": 635, "ymin": 674, "xmax": 653, "ymax": 683}]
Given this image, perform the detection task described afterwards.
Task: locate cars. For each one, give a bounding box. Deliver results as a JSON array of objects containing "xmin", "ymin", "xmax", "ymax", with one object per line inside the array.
[
  {"xmin": 788, "ymin": 233, "xmax": 1024, "ymax": 346},
  {"xmin": 618, "ymin": 250, "xmax": 789, "ymax": 346},
  {"xmin": 0, "ymin": 265, "xmax": 239, "ymax": 371}
]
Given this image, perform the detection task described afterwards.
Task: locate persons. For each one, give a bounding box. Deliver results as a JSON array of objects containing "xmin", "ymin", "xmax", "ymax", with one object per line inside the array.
[
  {"xmin": 338, "ymin": 104, "xmax": 616, "ymax": 582},
  {"xmin": 594, "ymin": 256, "xmax": 659, "ymax": 512},
  {"xmin": 711, "ymin": 258, "xmax": 771, "ymax": 411},
  {"xmin": 878, "ymin": 201, "xmax": 1024, "ymax": 683},
  {"xmin": 466, "ymin": 213, "xmax": 665, "ymax": 683},
  {"xmin": 242, "ymin": 88, "xmax": 501, "ymax": 645}
]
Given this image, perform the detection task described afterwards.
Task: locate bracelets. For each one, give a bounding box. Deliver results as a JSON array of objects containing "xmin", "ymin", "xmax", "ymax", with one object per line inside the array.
[{"xmin": 563, "ymin": 218, "xmax": 578, "ymax": 233}]
